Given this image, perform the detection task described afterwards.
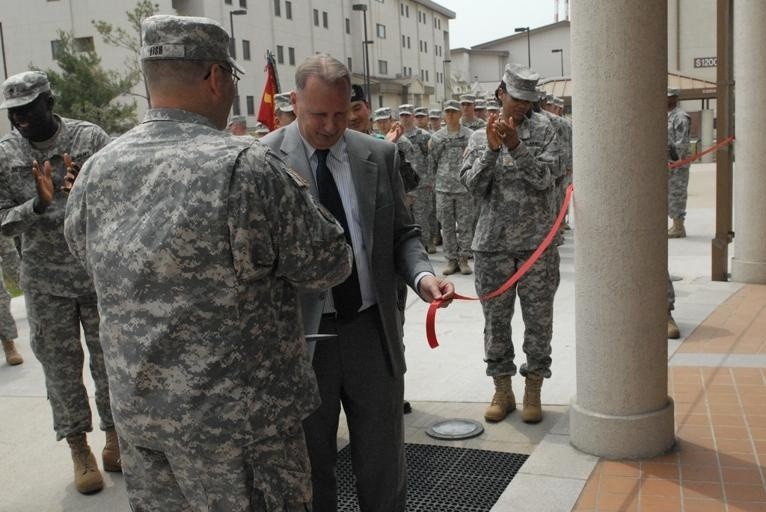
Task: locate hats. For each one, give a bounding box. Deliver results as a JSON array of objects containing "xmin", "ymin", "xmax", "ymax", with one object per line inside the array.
[
  {"xmin": 667, "ymin": 87, "xmax": 680, "ymax": 98},
  {"xmin": 228, "ymin": 114, "xmax": 271, "ymax": 136},
  {"xmin": 273, "ymin": 93, "xmax": 295, "ymax": 115},
  {"xmin": 350, "ymin": 84, "xmax": 367, "ymax": 102},
  {"xmin": 140, "ymin": 15, "xmax": 246, "ymax": 75},
  {"xmin": 373, "ymin": 63, "xmax": 564, "ymax": 122},
  {"xmin": 1, "ymin": 70, "xmax": 51, "ymax": 108}
]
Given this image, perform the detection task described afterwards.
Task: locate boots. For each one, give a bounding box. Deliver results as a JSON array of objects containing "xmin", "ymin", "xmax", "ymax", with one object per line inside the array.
[
  {"xmin": 101, "ymin": 429, "xmax": 130, "ymax": 472},
  {"xmin": 2, "ymin": 338, "xmax": 26, "ymax": 366},
  {"xmin": 668, "ymin": 217, "xmax": 687, "ymax": 239},
  {"xmin": 521, "ymin": 375, "xmax": 543, "ymax": 423},
  {"xmin": 65, "ymin": 433, "xmax": 105, "ymax": 495},
  {"xmin": 484, "ymin": 375, "xmax": 516, "ymax": 422}
]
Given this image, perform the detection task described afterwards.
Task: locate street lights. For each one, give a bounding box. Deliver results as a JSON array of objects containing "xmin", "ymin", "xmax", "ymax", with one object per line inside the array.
[
  {"xmin": 362, "ymin": 41, "xmax": 374, "ymax": 100},
  {"xmin": 230, "ymin": 10, "xmax": 247, "ymax": 116},
  {"xmin": 552, "ymin": 49, "xmax": 563, "ymax": 77},
  {"xmin": 443, "ymin": 60, "xmax": 452, "ymax": 102},
  {"xmin": 352, "ymin": 5, "xmax": 373, "ymax": 131},
  {"xmin": 514, "ymin": 26, "xmax": 531, "ymax": 69}
]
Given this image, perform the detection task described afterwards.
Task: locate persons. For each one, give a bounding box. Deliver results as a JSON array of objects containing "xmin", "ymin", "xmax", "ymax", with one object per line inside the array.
[{"xmin": 668, "ymin": 91, "xmax": 692, "ymax": 237}]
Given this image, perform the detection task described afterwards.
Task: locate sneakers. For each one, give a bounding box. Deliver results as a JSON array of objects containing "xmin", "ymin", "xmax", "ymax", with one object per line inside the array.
[
  {"xmin": 668, "ymin": 309, "xmax": 679, "ymax": 338},
  {"xmin": 558, "ymin": 221, "xmax": 571, "ymax": 234},
  {"xmin": 427, "ymin": 233, "xmax": 475, "ymax": 274}
]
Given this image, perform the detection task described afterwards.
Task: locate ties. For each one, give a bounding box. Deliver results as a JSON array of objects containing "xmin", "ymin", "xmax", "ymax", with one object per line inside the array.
[{"xmin": 315, "ymin": 148, "xmax": 364, "ymax": 318}]
